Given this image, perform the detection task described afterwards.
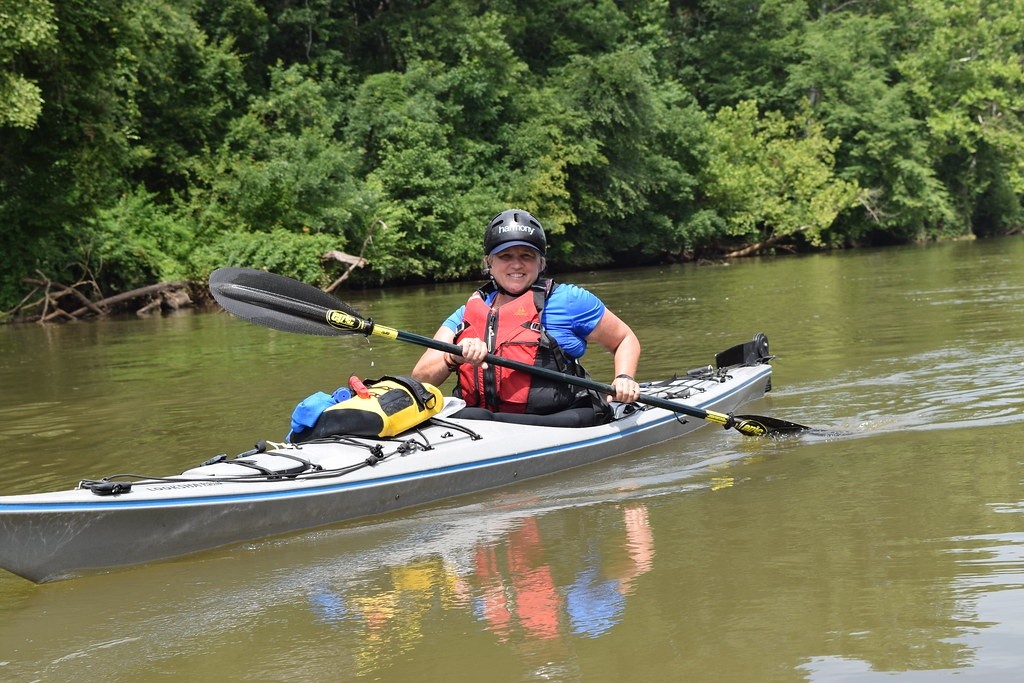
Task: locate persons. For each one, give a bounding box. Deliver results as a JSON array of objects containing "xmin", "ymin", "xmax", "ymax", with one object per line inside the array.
[
  {"xmin": 443, "ymin": 490, "xmax": 654, "ymax": 683},
  {"xmin": 411, "ymin": 209, "xmax": 641, "ymax": 428}
]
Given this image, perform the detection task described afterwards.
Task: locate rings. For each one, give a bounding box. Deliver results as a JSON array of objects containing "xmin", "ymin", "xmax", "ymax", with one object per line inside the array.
[
  {"xmin": 629, "ymin": 388, "xmax": 635, "ymax": 393},
  {"xmin": 469, "ymin": 341, "xmax": 475, "ymax": 345}
]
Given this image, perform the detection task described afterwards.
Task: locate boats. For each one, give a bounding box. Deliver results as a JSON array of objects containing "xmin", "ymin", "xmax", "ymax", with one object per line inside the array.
[{"xmin": 0, "ymin": 333, "xmax": 775, "ymax": 583}]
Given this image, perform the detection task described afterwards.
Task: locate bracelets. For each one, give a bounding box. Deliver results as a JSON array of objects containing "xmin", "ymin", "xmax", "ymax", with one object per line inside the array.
[{"xmin": 615, "ymin": 374, "xmax": 635, "ymax": 382}]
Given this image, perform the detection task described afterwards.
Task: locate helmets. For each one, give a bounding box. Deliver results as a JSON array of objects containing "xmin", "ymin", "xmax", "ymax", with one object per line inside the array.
[{"xmin": 483, "ymin": 208, "xmax": 546, "ymax": 257}]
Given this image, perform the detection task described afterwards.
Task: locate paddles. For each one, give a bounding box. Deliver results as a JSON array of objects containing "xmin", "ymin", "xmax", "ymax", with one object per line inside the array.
[{"xmin": 209, "ymin": 264, "xmax": 812, "ymax": 439}]
